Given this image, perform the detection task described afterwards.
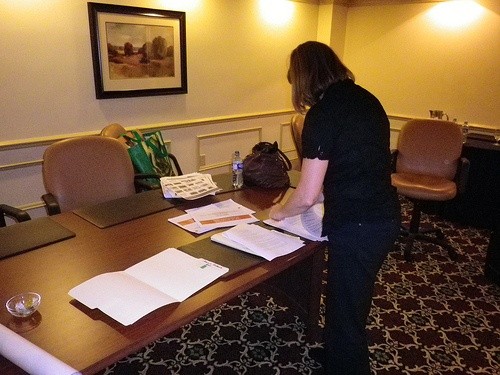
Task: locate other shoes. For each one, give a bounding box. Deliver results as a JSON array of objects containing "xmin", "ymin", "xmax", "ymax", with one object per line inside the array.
[{"xmin": 308, "ymin": 347, "xmax": 327, "ymax": 375}]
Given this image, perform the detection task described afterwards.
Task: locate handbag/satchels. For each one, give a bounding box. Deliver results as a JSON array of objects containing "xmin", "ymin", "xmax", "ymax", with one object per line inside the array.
[
  {"xmin": 242, "ymin": 140, "xmax": 294, "ymax": 192},
  {"xmin": 120, "ymin": 128, "xmax": 179, "ymax": 194}
]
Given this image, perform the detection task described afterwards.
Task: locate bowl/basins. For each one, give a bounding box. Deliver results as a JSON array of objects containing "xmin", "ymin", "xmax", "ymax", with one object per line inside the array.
[{"xmin": 5, "ymin": 293, "xmax": 41, "ymax": 317}]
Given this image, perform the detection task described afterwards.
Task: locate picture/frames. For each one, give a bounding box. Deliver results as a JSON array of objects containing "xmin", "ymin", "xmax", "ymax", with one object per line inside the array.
[{"xmin": 87, "ymin": 2, "xmax": 188, "ymax": 99}]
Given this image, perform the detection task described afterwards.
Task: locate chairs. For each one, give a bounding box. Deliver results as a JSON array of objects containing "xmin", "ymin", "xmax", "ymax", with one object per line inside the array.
[
  {"xmin": 42, "ymin": 124, "xmax": 183, "ymax": 216},
  {"xmin": 389, "ymin": 118, "xmax": 470, "ymax": 261},
  {"xmin": 290, "ymin": 113, "xmax": 304, "ymax": 161}
]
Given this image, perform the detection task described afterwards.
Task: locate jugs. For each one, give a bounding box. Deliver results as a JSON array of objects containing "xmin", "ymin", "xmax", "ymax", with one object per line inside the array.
[{"xmin": 429, "ymin": 110, "xmax": 448, "ymax": 120}]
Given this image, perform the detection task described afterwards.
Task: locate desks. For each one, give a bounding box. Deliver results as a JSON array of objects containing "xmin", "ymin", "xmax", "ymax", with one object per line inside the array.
[
  {"xmin": 0, "ymin": 169, "xmax": 326, "ymax": 375},
  {"xmin": 463, "ymin": 132, "xmax": 500, "ymax": 227}
]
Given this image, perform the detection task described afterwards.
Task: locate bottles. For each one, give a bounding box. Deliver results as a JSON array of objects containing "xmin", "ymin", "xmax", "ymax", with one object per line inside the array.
[
  {"xmin": 461, "ymin": 122, "xmax": 469, "ymax": 144},
  {"xmin": 232, "ymin": 151, "xmax": 243, "ymax": 188}
]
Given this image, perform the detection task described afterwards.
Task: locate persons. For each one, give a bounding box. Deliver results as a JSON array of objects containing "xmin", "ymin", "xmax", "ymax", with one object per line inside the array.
[{"xmin": 269, "ymin": 41, "xmax": 402, "ymax": 375}]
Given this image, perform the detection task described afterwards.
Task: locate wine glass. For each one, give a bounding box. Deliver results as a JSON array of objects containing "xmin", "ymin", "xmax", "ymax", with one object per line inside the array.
[{"xmin": 493, "ymin": 131, "xmax": 500, "ymax": 146}]
